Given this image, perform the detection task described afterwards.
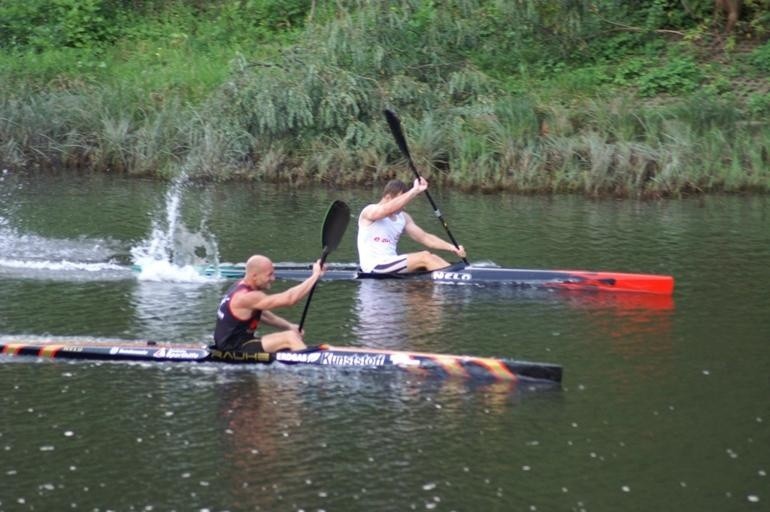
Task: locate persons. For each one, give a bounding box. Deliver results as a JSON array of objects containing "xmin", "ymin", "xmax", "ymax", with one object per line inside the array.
[
  {"xmin": 214, "ymin": 254, "xmax": 326, "ymax": 354},
  {"xmin": 356, "ymin": 176, "xmax": 466, "ymax": 275}
]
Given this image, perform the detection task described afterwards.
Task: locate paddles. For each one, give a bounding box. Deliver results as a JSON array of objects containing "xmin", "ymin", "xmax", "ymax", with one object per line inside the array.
[
  {"xmin": 383, "ymin": 107, "xmax": 469, "ymax": 267},
  {"xmin": 299, "ymin": 200, "xmax": 350, "ymax": 331}
]
